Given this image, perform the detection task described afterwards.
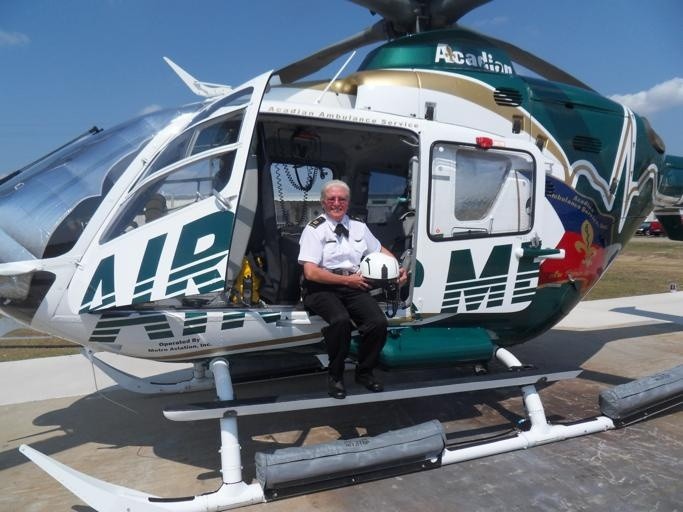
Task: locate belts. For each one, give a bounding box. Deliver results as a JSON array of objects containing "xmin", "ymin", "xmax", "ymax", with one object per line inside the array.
[{"xmin": 333, "ymin": 269, "xmax": 354, "ymax": 276}]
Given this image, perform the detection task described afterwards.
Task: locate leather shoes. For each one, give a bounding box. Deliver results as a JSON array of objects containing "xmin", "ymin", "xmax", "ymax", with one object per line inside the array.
[
  {"xmin": 328, "ymin": 376, "xmax": 345, "ymax": 399},
  {"xmin": 355, "ymin": 367, "xmax": 384, "ymax": 392}
]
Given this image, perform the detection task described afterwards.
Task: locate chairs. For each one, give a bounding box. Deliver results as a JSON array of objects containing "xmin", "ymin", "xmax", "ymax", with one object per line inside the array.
[{"xmin": 261, "ymin": 156, "xmax": 413, "ymax": 299}]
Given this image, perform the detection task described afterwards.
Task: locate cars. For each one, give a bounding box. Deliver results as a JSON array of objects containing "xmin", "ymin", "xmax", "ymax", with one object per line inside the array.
[{"xmin": 634, "ymin": 220, "xmax": 666, "ymax": 236}]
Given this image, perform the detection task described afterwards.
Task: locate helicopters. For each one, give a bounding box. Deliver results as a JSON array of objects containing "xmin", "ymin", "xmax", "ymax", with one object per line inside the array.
[{"xmin": 0, "ymin": 0, "xmax": 682, "ymax": 512}]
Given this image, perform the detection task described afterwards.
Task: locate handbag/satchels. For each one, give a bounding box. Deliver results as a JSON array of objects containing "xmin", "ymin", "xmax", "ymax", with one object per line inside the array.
[{"xmin": 258, "ymin": 236, "xmax": 299, "ymax": 304}]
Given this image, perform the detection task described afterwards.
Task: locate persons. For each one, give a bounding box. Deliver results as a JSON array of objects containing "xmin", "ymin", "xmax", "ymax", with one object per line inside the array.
[{"xmin": 295, "ymin": 178, "xmax": 409, "ymax": 400}]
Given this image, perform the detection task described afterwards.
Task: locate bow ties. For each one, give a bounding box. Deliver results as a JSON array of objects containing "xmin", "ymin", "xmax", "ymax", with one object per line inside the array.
[{"xmin": 335, "ymin": 224, "xmax": 348, "ymax": 238}]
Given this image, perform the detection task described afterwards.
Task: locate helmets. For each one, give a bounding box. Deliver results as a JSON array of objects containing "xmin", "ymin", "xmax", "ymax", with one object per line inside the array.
[{"xmin": 360, "ymin": 251, "xmax": 400, "ymax": 280}]
